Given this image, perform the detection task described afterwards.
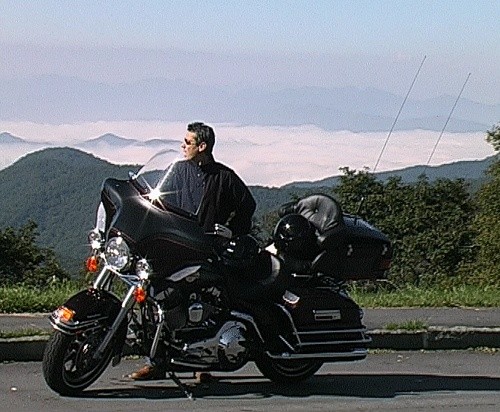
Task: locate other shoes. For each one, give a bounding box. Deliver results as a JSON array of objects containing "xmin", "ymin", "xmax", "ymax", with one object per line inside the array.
[
  {"xmin": 196, "ymin": 372, "xmax": 218, "ymax": 383},
  {"xmin": 128, "ymin": 364, "xmax": 165, "ymax": 381}
]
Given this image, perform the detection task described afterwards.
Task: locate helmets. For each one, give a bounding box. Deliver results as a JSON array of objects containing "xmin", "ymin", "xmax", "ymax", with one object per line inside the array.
[{"xmin": 273, "ymin": 213, "xmax": 316, "ymax": 258}]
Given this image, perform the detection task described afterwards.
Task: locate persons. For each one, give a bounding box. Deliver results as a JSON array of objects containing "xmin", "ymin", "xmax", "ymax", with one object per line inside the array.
[{"xmin": 122, "ymin": 121, "xmax": 257, "ymax": 388}]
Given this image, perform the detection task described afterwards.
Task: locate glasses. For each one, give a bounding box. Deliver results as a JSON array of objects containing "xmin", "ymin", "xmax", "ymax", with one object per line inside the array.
[{"xmin": 184, "ymin": 137, "xmax": 197, "ymax": 146}]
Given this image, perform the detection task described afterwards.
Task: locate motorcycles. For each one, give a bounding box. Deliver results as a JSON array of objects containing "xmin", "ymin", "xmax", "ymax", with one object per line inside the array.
[{"xmin": 39, "ymin": 148, "xmax": 394, "ymax": 402}]
